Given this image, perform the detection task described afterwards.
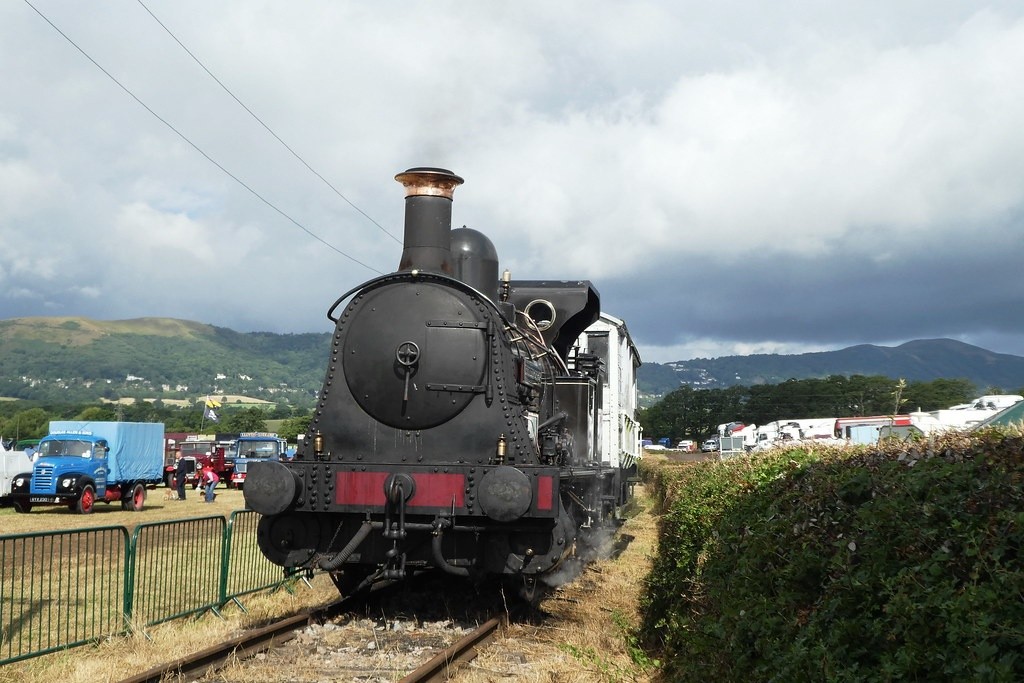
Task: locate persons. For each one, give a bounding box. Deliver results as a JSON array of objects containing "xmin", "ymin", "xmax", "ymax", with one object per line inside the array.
[
  {"xmin": 278, "ymin": 453, "xmax": 289, "ymax": 461},
  {"xmin": 198, "ymin": 468, "xmax": 219, "ymax": 503},
  {"xmin": 174, "ymin": 451, "xmax": 186, "ymax": 500}
]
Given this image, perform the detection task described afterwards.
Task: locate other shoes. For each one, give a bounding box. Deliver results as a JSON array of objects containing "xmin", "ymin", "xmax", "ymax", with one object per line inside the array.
[{"xmin": 176, "ymin": 497, "xmax": 185, "ymax": 500}]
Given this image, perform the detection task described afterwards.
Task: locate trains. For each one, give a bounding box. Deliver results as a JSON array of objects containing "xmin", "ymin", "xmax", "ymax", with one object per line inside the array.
[{"xmin": 241, "ymin": 163, "xmax": 644, "ymax": 618}]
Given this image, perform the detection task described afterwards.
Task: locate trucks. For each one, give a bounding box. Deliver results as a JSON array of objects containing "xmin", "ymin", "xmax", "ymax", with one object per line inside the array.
[
  {"xmin": 6, "ymin": 420, "xmax": 166, "ymax": 515},
  {"xmin": 163, "ymin": 431, "xmax": 296, "ymax": 491},
  {"xmin": 677, "ymin": 440, "xmax": 692, "ymax": 454},
  {"xmin": 658, "ymin": 438, "xmax": 670, "ymax": 449}
]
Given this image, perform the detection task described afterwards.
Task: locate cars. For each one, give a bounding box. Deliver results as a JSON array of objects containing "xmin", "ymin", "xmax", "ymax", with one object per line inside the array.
[
  {"xmin": 702, "ymin": 439, "xmax": 717, "ymax": 452},
  {"xmin": 0, "ymin": 438, "xmax": 42, "ymax": 468}
]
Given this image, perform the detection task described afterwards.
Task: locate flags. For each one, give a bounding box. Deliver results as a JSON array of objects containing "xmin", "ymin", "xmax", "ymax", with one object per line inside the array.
[
  {"xmin": 206, "ymin": 395, "xmax": 222, "ymax": 410},
  {"xmin": 204, "ymin": 405, "xmax": 222, "ymax": 424}
]
